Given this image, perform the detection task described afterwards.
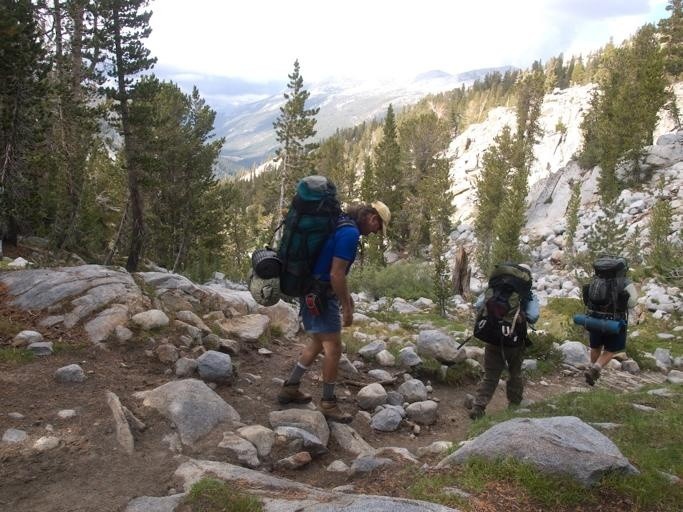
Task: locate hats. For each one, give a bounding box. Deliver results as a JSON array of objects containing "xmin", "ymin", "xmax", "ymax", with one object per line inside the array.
[{"xmin": 370, "ymin": 199, "xmax": 391, "ymax": 238}]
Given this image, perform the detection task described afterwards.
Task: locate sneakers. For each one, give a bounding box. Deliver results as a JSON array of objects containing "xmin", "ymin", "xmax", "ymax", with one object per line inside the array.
[
  {"xmin": 584, "ymin": 365, "xmax": 600, "ymax": 386},
  {"xmin": 277, "ymin": 380, "xmax": 313, "ymax": 405},
  {"xmin": 318, "ymin": 397, "xmax": 354, "ymax": 424},
  {"xmin": 470, "ymin": 404, "xmax": 485, "ymax": 420}
]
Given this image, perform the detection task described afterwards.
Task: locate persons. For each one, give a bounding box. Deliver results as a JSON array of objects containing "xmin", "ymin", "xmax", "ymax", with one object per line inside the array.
[
  {"xmin": 277, "ymin": 199, "xmax": 392, "ymax": 424},
  {"xmin": 468, "ymin": 263, "xmax": 541, "ymax": 421},
  {"xmin": 584, "ymin": 255, "xmax": 637, "ymax": 387}
]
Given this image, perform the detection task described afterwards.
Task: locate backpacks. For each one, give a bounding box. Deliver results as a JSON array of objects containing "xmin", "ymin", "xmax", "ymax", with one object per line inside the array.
[
  {"xmin": 583, "ymin": 258, "xmax": 630, "ymax": 321},
  {"xmin": 276, "ymin": 175, "xmax": 358, "ymax": 297},
  {"xmin": 473, "ymin": 261, "xmax": 533, "ymax": 349}
]
